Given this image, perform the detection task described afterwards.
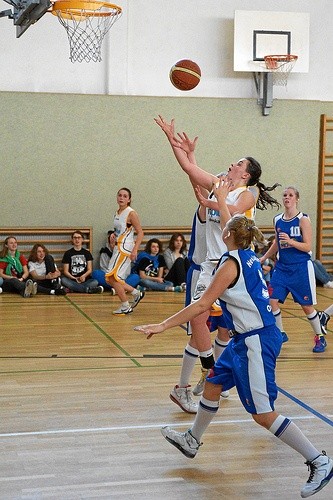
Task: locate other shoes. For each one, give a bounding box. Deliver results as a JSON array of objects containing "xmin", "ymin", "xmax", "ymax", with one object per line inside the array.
[
  {"xmin": 323, "ymin": 281, "xmax": 333, "ymax": 288},
  {"xmin": 137, "ymin": 285, "xmax": 145, "ymax": 290},
  {"xmin": 173, "ymin": 286, "xmax": 182, "ymax": 292},
  {"xmin": 24, "ymin": 279, "xmax": 33, "ymax": 297},
  {"xmin": 31, "ymin": 282, "xmax": 37, "ymax": 295},
  {"xmin": 89, "ymin": 286, "xmax": 104, "ymax": 294},
  {"xmin": 55, "ymin": 289, "xmax": 66, "ymax": 295},
  {"xmin": 111, "ymin": 288, "xmax": 118, "ymax": 296}
]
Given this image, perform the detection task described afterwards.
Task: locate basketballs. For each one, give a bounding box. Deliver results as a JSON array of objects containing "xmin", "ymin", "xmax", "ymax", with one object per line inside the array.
[{"xmin": 169, "ymin": 60, "xmax": 202, "ymax": 92}]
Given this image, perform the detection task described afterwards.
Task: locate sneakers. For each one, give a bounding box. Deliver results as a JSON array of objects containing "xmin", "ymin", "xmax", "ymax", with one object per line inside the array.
[
  {"xmin": 160, "ymin": 426, "xmax": 203, "ymax": 458},
  {"xmin": 301, "ymin": 450, "xmax": 333, "ymax": 497},
  {"xmin": 193, "ymin": 367, "xmax": 209, "ymax": 396},
  {"xmin": 113, "ymin": 305, "xmax": 133, "ymax": 315},
  {"xmin": 221, "ymin": 390, "xmax": 229, "ymax": 398},
  {"xmin": 313, "ymin": 334, "xmax": 327, "ymax": 352},
  {"xmin": 169, "ymin": 385, "xmax": 199, "ymax": 413},
  {"xmin": 318, "ymin": 311, "xmax": 330, "ymax": 334},
  {"xmin": 131, "ymin": 290, "xmax": 145, "ymax": 308},
  {"xmin": 281, "ymin": 331, "xmax": 288, "ymax": 343}
]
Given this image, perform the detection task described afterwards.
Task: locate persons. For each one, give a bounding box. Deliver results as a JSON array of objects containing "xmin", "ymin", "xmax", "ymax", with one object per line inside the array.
[
  {"xmin": 0, "ymin": 237, "xmax": 37, "ymax": 297},
  {"xmin": 133, "ymin": 214, "xmax": 333, "ymax": 498},
  {"xmin": 104, "ymin": 250, "xmax": 105, "ymax": 251},
  {"xmin": 258, "ymin": 186, "xmax": 327, "ymax": 352},
  {"xmin": 27, "ymin": 243, "xmax": 67, "ymax": 295},
  {"xmin": 62, "ymin": 231, "xmax": 104, "ymax": 294},
  {"xmin": 154, "ymin": 114, "xmax": 282, "ymax": 414},
  {"xmin": 267, "ymin": 236, "xmax": 333, "ymax": 288},
  {"xmin": 254, "ymin": 246, "xmax": 275, "ymax": 297},
  {"xmin": 135, "ymin": 238, "xmax": 183, "ymax": 292},
  {"xmin": 172, "ymin": 132, "xmax": 230, "ymax": 398},
  {"xmin": 92, "ymin": 230, "xmax": 141, "ymax": 295},
  {"xmin": 104, "ymin": 187, "xmax": 145, "ymax": 314},
  {"xmin": 318, "ymin": 304, "xmax": 333, "ymax": 334},
  {"xmin": 163, "ymin": 233, "xmax": 192, "ymax": 293}
]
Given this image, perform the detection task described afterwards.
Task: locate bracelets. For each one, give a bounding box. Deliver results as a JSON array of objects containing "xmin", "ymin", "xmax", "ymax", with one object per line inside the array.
[{"xmin": 22, "ymin": 277, "xmax": 25, "ymax": 281}]
